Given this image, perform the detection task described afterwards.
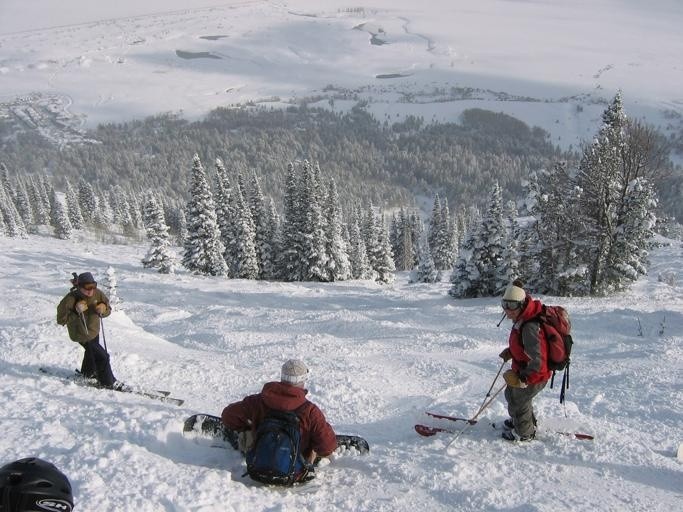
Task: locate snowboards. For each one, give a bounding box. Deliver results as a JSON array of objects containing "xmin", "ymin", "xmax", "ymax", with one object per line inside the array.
[{"xmin": 184, "ymin": 414, "xmax": 369, "ymax": 456}]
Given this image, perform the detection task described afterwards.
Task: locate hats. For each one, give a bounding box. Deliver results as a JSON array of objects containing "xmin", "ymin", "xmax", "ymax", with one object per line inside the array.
[
  {"xmin": 77, "ymin": 273, "xmax": 98, "ymax": 286},
  {"xmin": 281, "ymin": 358, "xmax": 308, "ymax": 384},
  {"xmin": 503, "ymin": 286, "xmax": 526, "ymax": 302}
]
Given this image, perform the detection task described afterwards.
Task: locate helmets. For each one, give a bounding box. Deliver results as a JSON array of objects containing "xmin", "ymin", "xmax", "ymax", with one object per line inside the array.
[{"xmin": 0, "ymin": 458, "xmax": 74, "ymax": 512}]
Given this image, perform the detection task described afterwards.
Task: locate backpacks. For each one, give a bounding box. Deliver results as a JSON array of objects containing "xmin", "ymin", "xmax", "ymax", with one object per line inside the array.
[
  {"xmin": 246, "ymin": 401, "xmax": 309, "ymax": 487},
  {"xmin": 521, "ymin": 304, "xmax": 572, "ymax": 370}
]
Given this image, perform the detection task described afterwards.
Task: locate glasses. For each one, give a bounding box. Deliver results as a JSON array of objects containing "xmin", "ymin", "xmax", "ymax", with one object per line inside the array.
[
  {"xmin": 500, "ymin": 300, "xmax": 520, "ymax": 310},
  {"xmin": 83, "ymin": 283, "xmax": 96, "ymax": 290}
]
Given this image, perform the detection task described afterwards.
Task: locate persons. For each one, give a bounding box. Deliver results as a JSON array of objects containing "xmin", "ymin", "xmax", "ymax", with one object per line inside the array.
[
  {"xmin": 499, "ymin": 286, "xmax": 555, "ymax": 441},
  {"xmin": 222, "ymin": 359, "xmax": 337, "ymax": 487},
  {"xmin": 57, "ymin": 273, "xmax": 125, "ymax": 391}
]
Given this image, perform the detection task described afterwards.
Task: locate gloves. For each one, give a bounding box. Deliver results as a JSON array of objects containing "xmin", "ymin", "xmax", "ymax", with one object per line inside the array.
[
  {"xmin": 95, "ymin": 301, "xmax": 107, "ymax": 314},
  {"xmin": 500, "ymin": 349, "xmax": 512, "ymax": 361},
  {"xmin": 503, "ymin": 370, "xmax": 520, "ymax": 385},
  {"xmin": 75, "ymin": 300, "xmax": 88, "ymax": 314}
]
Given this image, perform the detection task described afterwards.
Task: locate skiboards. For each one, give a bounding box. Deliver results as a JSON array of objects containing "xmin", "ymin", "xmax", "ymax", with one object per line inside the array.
[
  {"xmin": 40, "ymin": 367, "xmax": 183, "ymax": 407},
  {"xmin": 415, "ymin": 413, "xmax": 594, "ymax": 444}
]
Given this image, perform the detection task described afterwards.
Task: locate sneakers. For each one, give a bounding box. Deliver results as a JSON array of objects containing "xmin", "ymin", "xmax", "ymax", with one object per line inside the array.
[
  {"xmin": 501, "ymin": 415, "xmax": 536, "ymax": 440},
  {"xmin": 112, "ymin": 381, "xmax": 125, "ymax": 391},
  {"xmin": 81, "ymin": 368, "xmax": 96, "ymax": 377}
]
